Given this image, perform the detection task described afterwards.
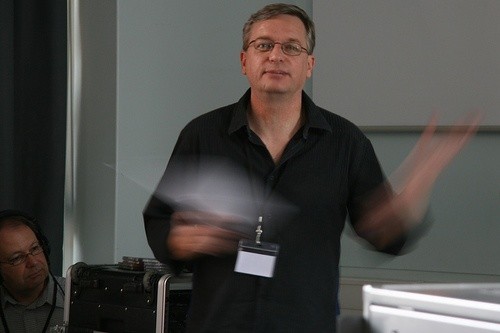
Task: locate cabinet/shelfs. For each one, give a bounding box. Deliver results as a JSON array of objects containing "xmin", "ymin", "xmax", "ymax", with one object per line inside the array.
[{"xmin": 361, "ymin": 280, "xmax": 500, "ymax": 331}]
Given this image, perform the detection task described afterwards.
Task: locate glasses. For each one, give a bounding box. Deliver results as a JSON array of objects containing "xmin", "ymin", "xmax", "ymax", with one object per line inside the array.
[
  {"xmin": 243, "ymin": 37, "xmax": 311, "ymax": 56},
  {"xmin": 0, "ymin": 246, "xmax": 43, "ymax": 266}
]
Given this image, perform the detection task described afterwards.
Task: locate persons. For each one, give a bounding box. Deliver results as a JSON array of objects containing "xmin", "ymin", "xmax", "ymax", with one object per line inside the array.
[
  {"xmin": 140, "ymin": 3, "xmax": 481, "ymax": 332},
  {"xmin": 1, "ymin": 207, "xmax": 66, "ymax": 333}
]
